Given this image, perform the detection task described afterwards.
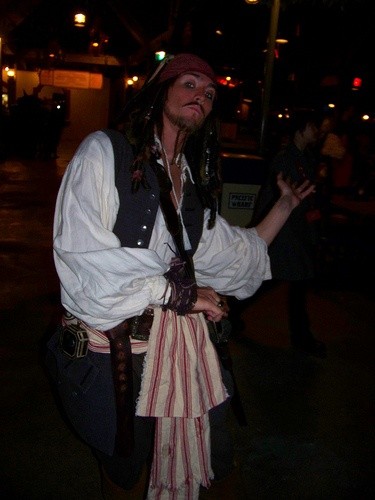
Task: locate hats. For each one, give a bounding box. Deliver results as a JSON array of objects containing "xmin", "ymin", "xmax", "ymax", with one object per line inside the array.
[{"xmin": 158, "ymin": 52, "xmax": 218, "ymax": 88}]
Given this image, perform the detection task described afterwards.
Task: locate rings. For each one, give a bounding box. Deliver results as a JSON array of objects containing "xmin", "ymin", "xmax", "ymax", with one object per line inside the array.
[{"xmin": 218, "ymin": 301, "xmax": 225, "ymax": 308}]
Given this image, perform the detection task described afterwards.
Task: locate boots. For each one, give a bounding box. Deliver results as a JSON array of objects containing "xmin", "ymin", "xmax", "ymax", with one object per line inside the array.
[
  {"xmin": 201, "ymin": 468, "xmax": 233, "ymax": 500},
  {"xmin": 107, "ymin": 462, "xmax": 146, "ymax": 500}
]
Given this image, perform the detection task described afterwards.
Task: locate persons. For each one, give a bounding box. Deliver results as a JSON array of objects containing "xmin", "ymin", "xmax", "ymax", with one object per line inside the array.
[
  {"xmin": 225, "ymin": 132, "xmax": 328, "ymax": 354},
  {"xmin": 8, "ymin": 90, "xmax": 63, "ymax": 161},
  {"xmin": 296, "ymin": 101, "xmax": 375, "ymax": 201},
  {"xmin": 42, "ymin": 52, "xmax": 315, "ymax": 500}
]
